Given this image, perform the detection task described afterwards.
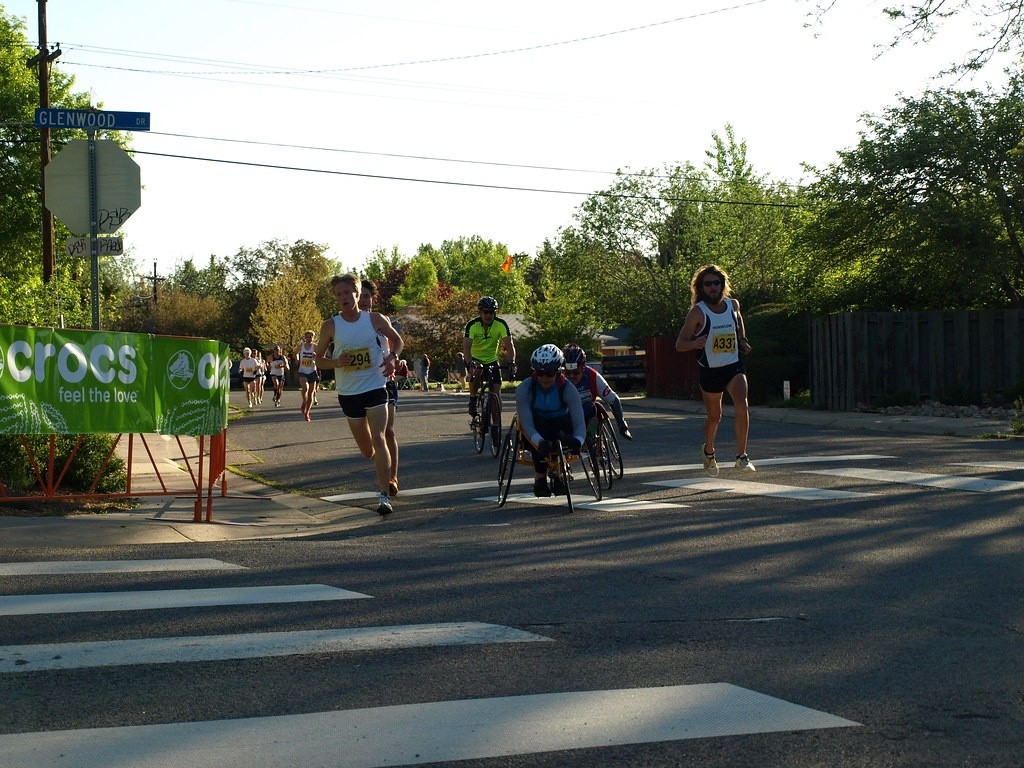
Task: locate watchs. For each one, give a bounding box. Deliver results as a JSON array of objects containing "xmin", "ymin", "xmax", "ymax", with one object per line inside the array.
[{"xmin": 390, "ymin": 352, "xmax": 398, "ymax": 358}]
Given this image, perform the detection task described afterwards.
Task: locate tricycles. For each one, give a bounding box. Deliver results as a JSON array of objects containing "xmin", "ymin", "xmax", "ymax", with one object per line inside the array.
[
  {"xmin": 585, "ymin": 402, "xmax": 624, "ymax": 491},
  {"xmin": 497, "ymin": 412, "xmax": 603, "ymax": 515}
]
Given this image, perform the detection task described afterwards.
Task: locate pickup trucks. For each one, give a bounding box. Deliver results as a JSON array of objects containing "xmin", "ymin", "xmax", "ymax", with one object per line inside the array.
[{"xmin": 601, "ymin": 354, "xmax": 648, "ymax": 393}]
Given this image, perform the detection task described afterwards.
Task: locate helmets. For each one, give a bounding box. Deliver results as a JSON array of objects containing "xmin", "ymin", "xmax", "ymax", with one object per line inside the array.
[
  {"xmin": 478, "ymin": 296, "xmax": 499, "ymax": 311},
  {"xmin": 530, "ymin": 343, "xmax": 566, "ymax": 370},
  {"xmin": 562, "ymin": 344, "xmax": 586, "ymax": 371}
]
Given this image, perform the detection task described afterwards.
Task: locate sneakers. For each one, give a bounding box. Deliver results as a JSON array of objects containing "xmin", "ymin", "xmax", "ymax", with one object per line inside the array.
[
  {"xmin": 701, "ymin": 442, "xmax": 720, "ymax": 478},
  {"xmin": 389, "ymin": 478, "xmax": 398, "ymax": 496},
  {"xmin": 377, "ymin": 494, "xmax": 394, "ymax": 515},
  {"xmin": 734, "ymin": 453, "xmax": 756, "ymax": 472}
]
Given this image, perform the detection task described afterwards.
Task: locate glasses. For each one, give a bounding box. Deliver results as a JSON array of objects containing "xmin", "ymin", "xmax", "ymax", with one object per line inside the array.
[
  {"xmin": 703, "ymin": 279, "xmax": 723, "ymax": 288},
  {"xmin": 563, "ymin": 367, "xmax": 583, "ymax": 376},
  {"xmin": 535, "ymin": 370, "xmax": 558, "ymax": 378},
  {"xmin": 481, "ymin": 309, "xmax": 496, "ymax": 315}
]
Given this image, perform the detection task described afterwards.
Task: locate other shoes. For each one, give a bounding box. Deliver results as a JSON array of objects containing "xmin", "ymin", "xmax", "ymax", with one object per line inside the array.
[
  {"xmin": 305, "ymin": 414, "xmax": 310, "ymax": 421},
  {"xmin": 469, "ymin": 399, "xmax": 478, "ymax": 417},
  {"xmin": 275, "ymin": 399, "xmax": 280, "ymax": 407},
  {"xmin": 248, "ymin": 402, "xmax": 253, "ymax": 408},
  {"xmin": 301, "ymin": 401, "xmax": 307, "ymax": 414},
  {"xmin": 553, "ymin": 477, "xmax": 568, "ymax": 494},
  {"xmin": 533, "ymin": 477, "xmax": 551, "ymax": 497},
  {"xmin": 491, "ymin": 430, "xmax": 502, "ymax": 446},
  {"xmin": 422, "ymin": 389, "xmax": 428, "ymax": 392},
  {"xmin": 273, "ymin": 394, "xmax": 276, "ymax": 402}
]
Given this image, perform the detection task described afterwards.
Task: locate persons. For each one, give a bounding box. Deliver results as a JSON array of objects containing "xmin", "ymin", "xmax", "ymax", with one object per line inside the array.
[
  {"xmin": 292, "ymin": 330, "xmax": 318, "ymax": 421},
  {"xmin": 456, "ymin": 352, "xmax": 467, "ymax": 390},
  {"xmin": 675, "ymin": 264, "xmax": 756, "ymax": 476},
  {"xmin": 462, "ymin": 295, "xmax": 517, "ymax": 439},
  {"xmin": 239, "ymin": 347, "xmax": 325, "ymax": 408},
  {"xmin": 314, "ymin": 273, "xmax": 409, "ymax": 513},
  {"xmin": 516, "ymin": 344, "xmax": 586, "ymax": 497},
  {"xmin": 420, "ymin": 354, "xmax": 431, "ymax": 391},
  {"xmin": 558, "ymin": 344, "xmax": 633, "ymax": 451}
]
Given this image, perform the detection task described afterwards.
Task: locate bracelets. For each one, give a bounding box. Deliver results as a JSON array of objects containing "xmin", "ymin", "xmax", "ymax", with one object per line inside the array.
[{"xmin": 739, "ymin": 338, "xmax": 749, "ymax": 343}]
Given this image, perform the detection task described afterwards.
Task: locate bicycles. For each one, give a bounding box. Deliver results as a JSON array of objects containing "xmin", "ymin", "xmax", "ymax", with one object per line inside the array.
[{"xmin": 467, "ymin": 361, "xmax": 517, "ymax": 459}]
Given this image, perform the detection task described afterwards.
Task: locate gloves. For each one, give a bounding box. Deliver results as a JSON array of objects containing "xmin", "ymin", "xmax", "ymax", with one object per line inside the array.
[
  {"xmin": 618, "ymin": 420, "xmax": 633, "ymax": 441},
  {"xmin": 539, "ymin": 441, "xmax": 554, "ymax": 453},
  {"xmin": 566, "ymin": 437, "xmax": 581, "ymax": 451}
]
